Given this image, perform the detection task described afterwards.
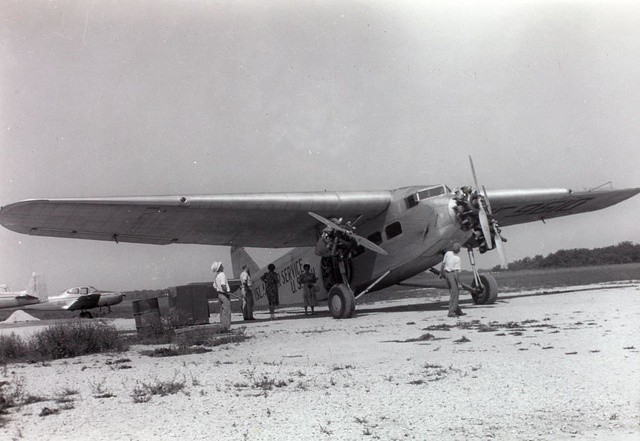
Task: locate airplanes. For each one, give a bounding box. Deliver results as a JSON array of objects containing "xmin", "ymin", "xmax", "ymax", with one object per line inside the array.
[
  {"xmin": 0, "ymin": 271, "xmax": 49, "ymax": 310},
  {"xmin": 0, "ymin": 285, "xmax": 128, "ymax": 319},
  {"xmin": 0, "ymin": 154, "xmax": 640, "ymax": 319}
]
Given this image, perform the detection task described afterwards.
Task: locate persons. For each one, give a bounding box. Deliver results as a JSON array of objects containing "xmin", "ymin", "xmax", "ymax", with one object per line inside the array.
[
  {"xmin": 259, "ymin": 264, "xmax": 280, "ymax": 320},
  {"xmin": 297, "ymin": 264, "xmax": 317, "ymax": 317},
  {"xmin": 210, "ymin": 262, "xmax": 233, "ymax": 334},
  {"xmin": 239, "ymin": 264, "xmax": 254, "ymax": 321},
  {"xmin": 439, "ymin": 243, "xmax": 467, "ymax": 318}
]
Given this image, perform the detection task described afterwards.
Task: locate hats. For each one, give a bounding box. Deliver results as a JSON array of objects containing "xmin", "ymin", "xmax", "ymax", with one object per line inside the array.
[{"xmin": 211, "ymin": 262, "xmax": 222, "ymax": 273}]
